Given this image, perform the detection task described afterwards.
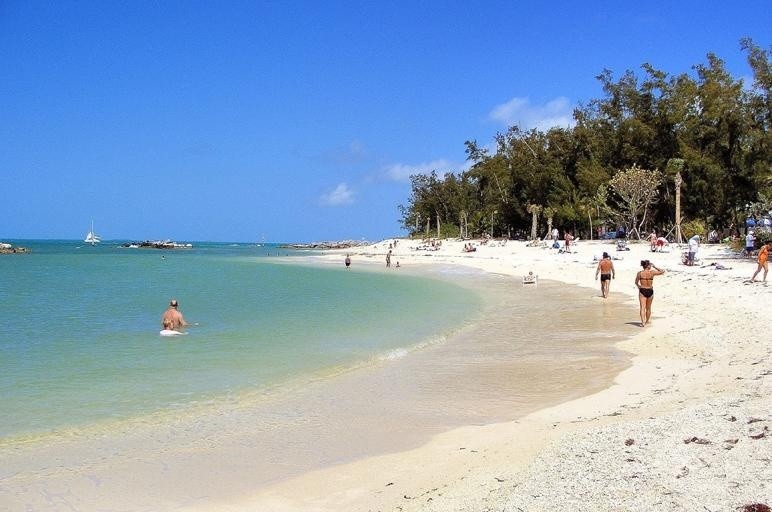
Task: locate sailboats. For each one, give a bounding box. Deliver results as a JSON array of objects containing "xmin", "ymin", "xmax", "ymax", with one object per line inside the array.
[{"xmin": 84, "ymin": 231, "xmax": 101, "ymax": 243}]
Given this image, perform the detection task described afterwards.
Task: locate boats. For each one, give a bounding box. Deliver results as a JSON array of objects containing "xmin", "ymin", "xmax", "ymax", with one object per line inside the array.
[
  {"xmin": 0, "ymin": 242, "xmax": 32, "ymax": 254},
  {"xmin": 117, "ymin": 239, "xmax": 192, "ymax": 248}
]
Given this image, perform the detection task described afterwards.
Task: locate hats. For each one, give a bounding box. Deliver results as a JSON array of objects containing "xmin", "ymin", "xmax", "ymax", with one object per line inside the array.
[{"xmin": 748, "ymin": 231, "xmax": 754, "ymax": 235}]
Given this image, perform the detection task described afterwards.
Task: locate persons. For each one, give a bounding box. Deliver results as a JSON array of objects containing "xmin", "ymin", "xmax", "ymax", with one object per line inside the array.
[
  {"xmin": 462, "ymin": 233, "xmax": 490, "ymax": 251},
  {"xmin": 745, "ymin": 230, "xmax": 757, "ymax": 259},
  {"xmin": 687, "ymin": 235, "xmax": 699, "ymax": 266},
  {"xmin": 564, "ymin": 233, "xmax": 573, "ymax": 251},
  {"xmin": 645, "ymin": 228, "xmax": 670, "ymax": 252},
  {"xmin": 163, "ymin": 299, "xmax": 188, "ymax": 330},
  {"xmin": 618, "ymin": 225, "xmax": 625, "ymax": 238},
  {"xmin": 750, "ymin": 241, "xmax": 772, "ymax": 284},
  {"xmin": 529, "ymin": 233, "xmax": 541, "ymax": 246},
  {"xmin": 595, "ymin": 252, "xmax": 615, "ymax": 298},
  {"xmin": 551, "ymin": 226, "xmax": 559, "ymax": 242},
  {"xmin": 635, "ymin": 259, "xmax": 664, "ymax": 327},
  {"xmin": 525, "ymin": 271, "xmax": 535, "ymax": 281},
  {"xmin": 345, "ymin": 254, "xmax": 351, "ymax": 270},
  {"xmin": 385, "ymin": 235, "xmax": 442, "ymax": 268}
]
